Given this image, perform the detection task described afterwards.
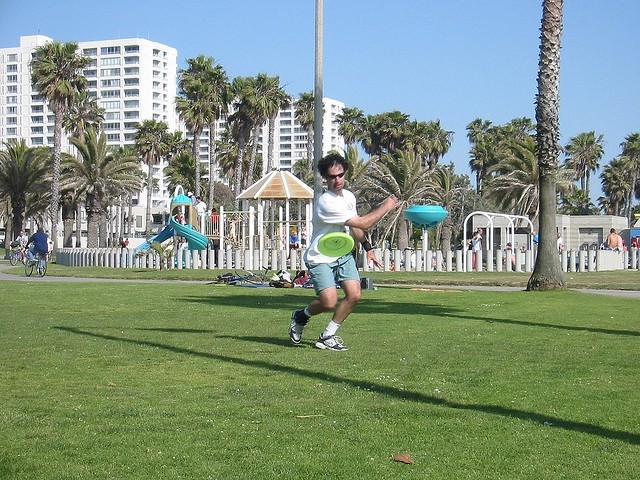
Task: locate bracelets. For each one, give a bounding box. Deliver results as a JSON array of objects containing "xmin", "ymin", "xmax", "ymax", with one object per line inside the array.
[{"xmin": 361, "ymin": 240, "xmax": 371, "ymax": 250}]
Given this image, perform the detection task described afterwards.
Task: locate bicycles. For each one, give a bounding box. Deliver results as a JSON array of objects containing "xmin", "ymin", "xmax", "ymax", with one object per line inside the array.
[
  {"xmin": 25, "ymin": 247, "xmax": 48, "ymax": 277},
  {"xmin": 10, "ymin": 242, "xmax": 30, "ymax": 266},
  {"xmin": 206, "ymin": 265, "xmax": 272, "ymax": 286}
]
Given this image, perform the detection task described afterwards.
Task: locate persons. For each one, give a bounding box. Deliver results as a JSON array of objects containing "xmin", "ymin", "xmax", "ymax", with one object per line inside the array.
[
  {"xmin": 289, "ymin": 153, "xmax": 400, "ymax": 352},
  {"xmin": 505, "ymin": 243, "xmax": 514, "ymax": 254},
  {"xmin": 522, "ymin": 246, "xmax": 525, "ymax": 252},
  {"xmin": 294, "ymin": 270, "xmax": 308, "ymax": 283},
  {"xmin": 556, "ymin": 233, "xmax": 564, "ymax": 254},
  {"xmin": 467, "ymin": 228, "xmax": 484, "ymax": 271},
  {"xmin": 622, "ymin": 240, "xmax": 628, "ymax": 250},
  {"xmin": 25, "ymin": 227, "xmax": 49, "ymax": 270},
  {"xmin": 124, "ymin": 237, "xmax": 130, "ymax": 247},
  {"xmin": 601, "ymin": 238, "xmax": 608, "ymax": 250},
  {"xmin": 300, "ymin": 227, "xmax": 306, "ymax": 247},
  {"xmin": 602, "ymin": 228, "xmax": 624, "ymax": 252},
  {"xmin": 211, "ymin": 207, "xmax": 218, "ymax": 224},
  {"xmin": 291, "ymin": 270, "xmax": 300, "ymax": 283},
  {"xmin": 195, "ymin": 195, "xmax": 206, "ymax": 216},
  {"xmin": 187, "ymin": 192, "xmax": 196, "ymax": 206},
  {"xmin": 631, "ymin": 235, "xmax": 637, "ymax": 247},
  {"xmin": 178, "ymin": 212, "xmax": 185, "ymax": 226},
  {"xmin": 289, "ymin": 229, "xmax": 298, "ymax": 248},
  {"xmin": 16, "ymin": 230, "xmax": 28, "ymax": 261}
]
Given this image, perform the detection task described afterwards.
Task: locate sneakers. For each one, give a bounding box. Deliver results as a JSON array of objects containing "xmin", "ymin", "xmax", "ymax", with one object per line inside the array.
[
  {"xmin": 315, "ymin": 333, "xmax": 347, "ymax": 351},
  {"xmin": 289, "ymin": 309, "xmax": 304, "ymax": 343},
  {"xmin": 27, "ymin": 260, "xmax": 37, "ymax": 267}
]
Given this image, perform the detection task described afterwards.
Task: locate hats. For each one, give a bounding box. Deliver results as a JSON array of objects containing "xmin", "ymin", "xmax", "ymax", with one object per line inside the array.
[{"xmin": 188, "ymin": 192, "xmax": 193, "ymax": 197}]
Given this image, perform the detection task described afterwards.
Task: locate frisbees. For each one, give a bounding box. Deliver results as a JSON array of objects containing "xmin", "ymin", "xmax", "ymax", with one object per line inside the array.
[{"xmin": 318, "ymin": 231, "xmax": 355, "ymax": 257}]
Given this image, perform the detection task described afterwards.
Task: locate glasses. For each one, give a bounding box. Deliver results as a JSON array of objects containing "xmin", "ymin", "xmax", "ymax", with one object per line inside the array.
[{"xmin": 325, "ymin": 171, "xmax": 345, "ymax": 178}]
